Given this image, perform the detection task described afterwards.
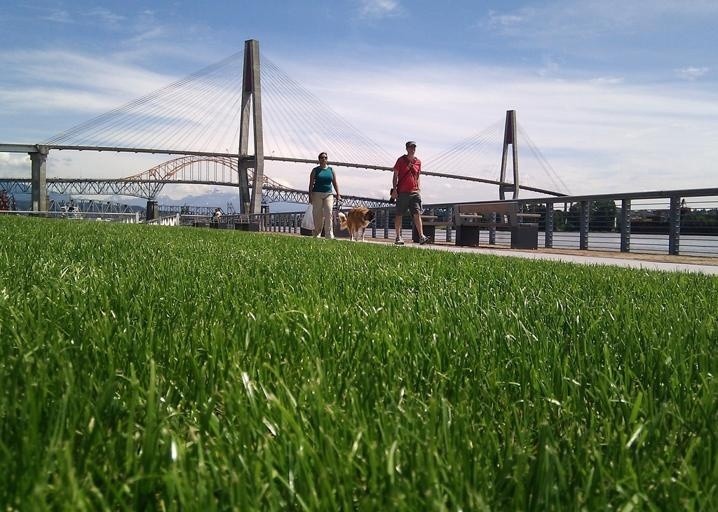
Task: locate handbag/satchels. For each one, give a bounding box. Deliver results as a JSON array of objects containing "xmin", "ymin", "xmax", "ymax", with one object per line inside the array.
[
  {"xmin": 389, "ymin": 188, "xmax": 399, "ymax": 203},
  {"xmin": 301, "ymin": 203, "xmax": 315, "ymax": 232}
]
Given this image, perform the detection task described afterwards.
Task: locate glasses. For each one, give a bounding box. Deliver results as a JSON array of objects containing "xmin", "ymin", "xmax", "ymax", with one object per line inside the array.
[{"xmin": 320, "ymin": 157, "xmax": 327, "ymax": 159}]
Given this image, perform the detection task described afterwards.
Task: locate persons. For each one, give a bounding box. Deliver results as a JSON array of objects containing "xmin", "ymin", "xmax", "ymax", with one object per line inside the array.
[
  {"xmin": 211, "ymin": 207, "xmax": 222, "ymax": 223},
  {"xmin": 309, "ymin": 152, "xmax": 342, "ymax": 240},
  {"xmin": 393, "ymin": 140, "xmax": 429, "ymax": 245}
]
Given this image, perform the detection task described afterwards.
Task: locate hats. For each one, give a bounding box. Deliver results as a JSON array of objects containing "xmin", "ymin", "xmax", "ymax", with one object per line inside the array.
[{"xmin": 406, "ymin": 141, "xmax": 417, "ymax": 147}]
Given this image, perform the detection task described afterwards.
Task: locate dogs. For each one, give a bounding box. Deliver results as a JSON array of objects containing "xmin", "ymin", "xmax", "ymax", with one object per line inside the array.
[{"xmin": 338, "ymin": 207, "xmax": 375, "ymax": 243}]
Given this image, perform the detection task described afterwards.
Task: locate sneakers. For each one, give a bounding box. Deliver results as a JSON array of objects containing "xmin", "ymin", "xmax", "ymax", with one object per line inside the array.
[
  {"xmin": 395, "ymin": 237, "xmax": 404, "ymax": 245},
  {"xmin": 419, "ymin": 236, "xmax": 430, "ymax": 244}
]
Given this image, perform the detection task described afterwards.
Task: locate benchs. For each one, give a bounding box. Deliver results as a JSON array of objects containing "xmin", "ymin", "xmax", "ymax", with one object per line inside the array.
[{"xmin": 452, "ymin": 199, "xmax": 540, "ymax": 249}]
